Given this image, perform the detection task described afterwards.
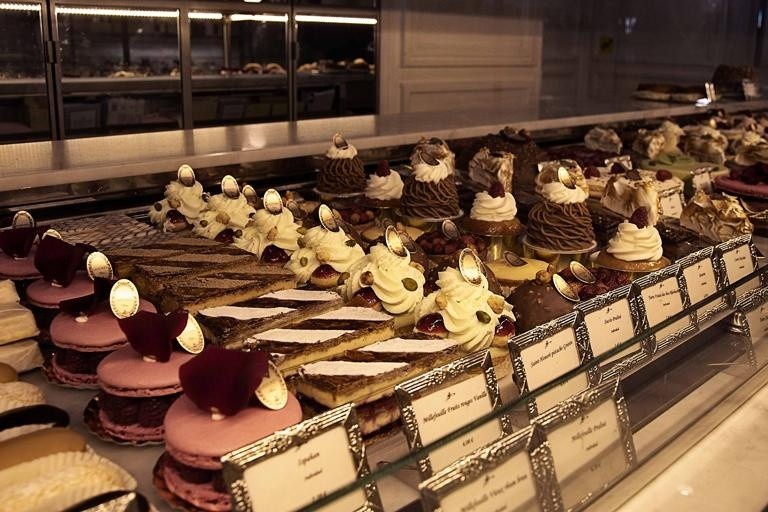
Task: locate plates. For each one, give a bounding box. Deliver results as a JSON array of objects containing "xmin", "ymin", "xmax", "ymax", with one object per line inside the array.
[
  {"xmin": 41, "ymin": 362, "xmax": 99, "ymax": 391},
  {"xmin": 79, "ymin": 395, "xmax": 166, "ymax": 448},
  {"xmin": 153, "ymin": 449, "xmax": 231, "ymax": 511}
]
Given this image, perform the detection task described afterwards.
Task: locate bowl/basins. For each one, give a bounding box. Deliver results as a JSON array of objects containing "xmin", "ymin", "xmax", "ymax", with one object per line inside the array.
[
  {"xmin": 587, "ymin": 251, "xmax": 672, "ymax": 289},
  {"xmin": 355, "ymin": 198, "xmax": 393, "ymax": 217},
  {"xmin": 455, "ymin": 223, "xmax": 526, "ymax": 263},
  {"xmin": 520, "ymin": 235, "xmax": 599, "ymax": 270},
  {"xmin": 395, "ymin": 207, "xmax": 466, "ymax": 231},
  {"xmin": 313, "ymin": 188, "xmax": 365, "ymax": 211}
]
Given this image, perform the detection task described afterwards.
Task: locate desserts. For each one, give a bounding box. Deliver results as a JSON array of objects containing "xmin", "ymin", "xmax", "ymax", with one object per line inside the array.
[{"xmin": 0, "ymin": 63, "xmax": 768, "ymax": 512}]
